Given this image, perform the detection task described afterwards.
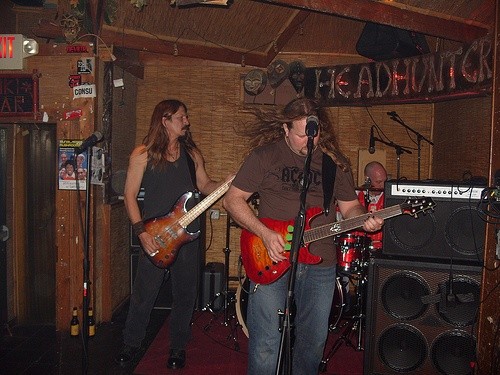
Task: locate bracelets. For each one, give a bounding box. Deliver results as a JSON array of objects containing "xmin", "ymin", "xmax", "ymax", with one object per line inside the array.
[{"xmin": 132, "ymin": 221, "xmax": 146, "ymax": 236}]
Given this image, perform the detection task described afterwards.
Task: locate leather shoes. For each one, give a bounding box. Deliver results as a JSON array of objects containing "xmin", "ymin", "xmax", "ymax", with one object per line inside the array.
[
  {"xmin": 118, "ymin": 352, "xmax": 130, "ymax": 362},
  {"xmin": 168, "ymin": 350, "xmax": 186, "ymax": 369}
]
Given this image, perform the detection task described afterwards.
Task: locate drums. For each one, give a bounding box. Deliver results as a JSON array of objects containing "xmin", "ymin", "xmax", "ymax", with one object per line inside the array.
[
  {"xmin": 234, "ymin": 272, "xmax": 346, "ymax": 342},
  {"xmin": 332, "ymin": 233, "xmax": 374, "ymax": 276}
]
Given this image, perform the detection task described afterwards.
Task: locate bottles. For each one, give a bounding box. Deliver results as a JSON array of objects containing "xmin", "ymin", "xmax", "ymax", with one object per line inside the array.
[
  {"xmin": 70, "ymin": 306, "xmax": 79, "ymax": 336},
  {"xmin": 87, "ymin": 305, "xmax": 96, "ymax": 337}
]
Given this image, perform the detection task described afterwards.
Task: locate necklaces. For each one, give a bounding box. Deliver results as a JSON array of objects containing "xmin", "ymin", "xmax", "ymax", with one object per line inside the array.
[{"xmin": 168, "ymin": 143, "xmax": 178, "ymax": 161}]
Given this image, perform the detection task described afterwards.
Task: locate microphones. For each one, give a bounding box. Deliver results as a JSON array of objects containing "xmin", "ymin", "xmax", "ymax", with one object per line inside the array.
[
  {"xmin": 369, "ymin": 127, "xmax": 375, "ymax": 154},
  {"xmin": 387, "ymin": 112, "xmax": 398, "ymax": 116},
  {"xmin": 306, "ymin": 116, "xmax": 319, "ymax": 138},
  {"xmin": 74, "ymin": 131, "xmax": 103, "ymax": 154}
]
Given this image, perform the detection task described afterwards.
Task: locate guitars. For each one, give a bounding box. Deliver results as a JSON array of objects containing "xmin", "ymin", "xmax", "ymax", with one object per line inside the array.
[
  {"xmin": 138, "ymin": 173, "xmax": 236, "ymax": 270},
  {"xmin": 240, "ymin": 195, "xmax": 438, "ymax": 286}
]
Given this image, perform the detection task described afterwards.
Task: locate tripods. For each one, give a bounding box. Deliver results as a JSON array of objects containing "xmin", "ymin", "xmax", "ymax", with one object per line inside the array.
[{"xmin": 190, "ymin": 213, "xmax": 245, "ymax": 351}]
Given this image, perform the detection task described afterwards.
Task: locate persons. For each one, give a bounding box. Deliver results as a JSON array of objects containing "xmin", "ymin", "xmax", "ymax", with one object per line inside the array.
[
  {"xmin": 335, "ymin": 161, "xmax": 389, "ymax": 223},
  {"xmin": 113, "ymin": 100, "xmax": 236, "ymax": 369},
  {"xmin": 59, "ymin": 153, "xmax": 87, "ymax": 180},
  {"xmin": 222, "ymin": 97, "xmax": 384, "ymax": 375}
]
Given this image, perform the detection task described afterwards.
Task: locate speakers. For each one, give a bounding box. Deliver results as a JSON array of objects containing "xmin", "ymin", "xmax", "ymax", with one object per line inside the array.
[
  {"xmin": 363, "ymin": 257, "xmax": 484, "ymax": 375},
  {"xmin": 382, "ymin": 179, "xmax": 487, "ymax": 263},
  {"xmin": 356, "ymin": 21, "xmax": 430, "ymax": 61}
]
